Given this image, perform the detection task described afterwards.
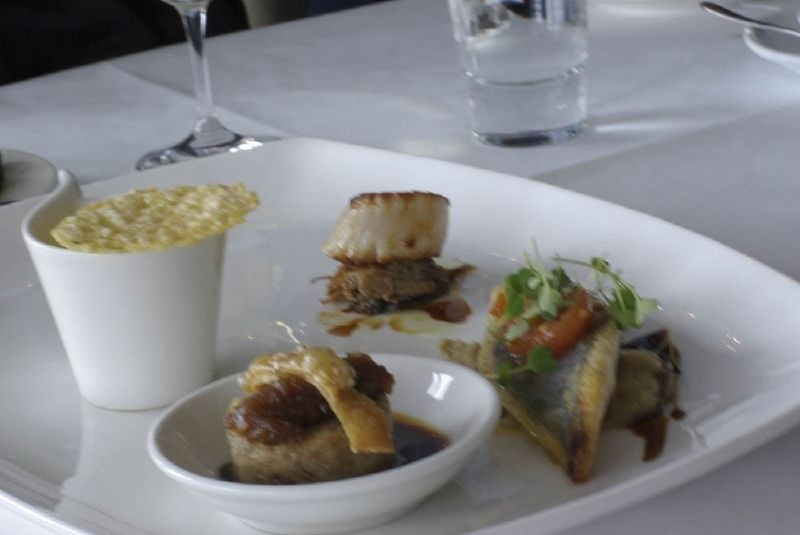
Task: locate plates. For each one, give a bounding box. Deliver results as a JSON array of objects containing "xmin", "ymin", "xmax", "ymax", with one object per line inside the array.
[
  {"xmin": 743, "ymin": 15, "xmax": 800, "ymax": 71},
  {"xmin": 0, "ymin": 135, "xmax": 800, "ymax": 535}
]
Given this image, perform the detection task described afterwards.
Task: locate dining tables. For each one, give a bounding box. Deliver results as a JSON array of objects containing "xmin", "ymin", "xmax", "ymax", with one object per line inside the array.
[{"xmin": 0, "ymin": 0, "xmax": 800, "ymax": 535}]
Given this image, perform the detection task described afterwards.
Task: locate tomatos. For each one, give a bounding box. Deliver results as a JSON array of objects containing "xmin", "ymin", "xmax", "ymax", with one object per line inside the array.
[{"xmin": 494, "ymin": 280, "xmax": 593, "ymax": 362}]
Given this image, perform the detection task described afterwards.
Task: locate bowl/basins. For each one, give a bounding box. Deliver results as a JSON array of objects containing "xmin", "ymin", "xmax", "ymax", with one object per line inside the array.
[{"xmin": 142, "ymin": 350, "xmax": 503, "ymax": 533}]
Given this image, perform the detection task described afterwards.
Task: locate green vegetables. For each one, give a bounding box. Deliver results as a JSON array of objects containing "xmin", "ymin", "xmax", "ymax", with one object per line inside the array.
[{"xmin": 489, "ymin": 239, "xmax": 662, "ymax": 382}]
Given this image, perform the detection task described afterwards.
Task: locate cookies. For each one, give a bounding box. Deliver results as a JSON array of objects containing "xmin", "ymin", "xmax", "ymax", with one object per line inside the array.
[{"xmin": 50, "ymin": 180, "xmax": 259, "ymax": 253}]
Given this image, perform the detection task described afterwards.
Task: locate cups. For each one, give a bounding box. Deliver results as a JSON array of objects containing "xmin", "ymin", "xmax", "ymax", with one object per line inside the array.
[
  {"xmin": 21, "ymin": 168, "xmax": 227, "ymax": 412},
  {"xmin": 447, "ymin": 0, "xmax": 592, "ymax": 147}
]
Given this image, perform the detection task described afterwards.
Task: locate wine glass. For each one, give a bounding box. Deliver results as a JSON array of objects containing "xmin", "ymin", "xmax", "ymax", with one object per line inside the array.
[{"xmin": 136, "ymin": 0, "xmax": 284, "ymax": 172}]
